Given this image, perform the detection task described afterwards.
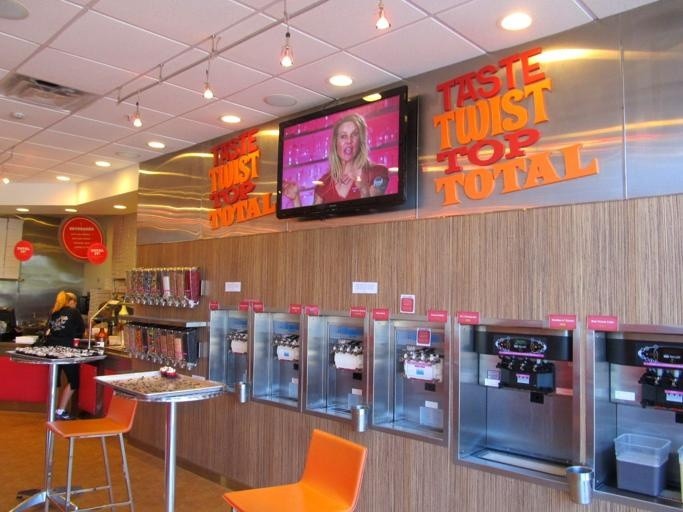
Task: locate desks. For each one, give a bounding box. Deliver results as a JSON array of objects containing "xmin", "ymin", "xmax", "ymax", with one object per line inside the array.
[{"xmin": 99, "ymin": 368, "xmax": 229, "ymax": 512}]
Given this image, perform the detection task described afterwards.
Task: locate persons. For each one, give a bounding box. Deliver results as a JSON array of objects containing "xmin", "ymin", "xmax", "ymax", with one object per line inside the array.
[
  {"xmin": 45, "ymin": 290, "xmax": 80, "ymax": 419},
  {"xmin": 283, "ymin": 113, "xmax": 389, "ymax": 208}
]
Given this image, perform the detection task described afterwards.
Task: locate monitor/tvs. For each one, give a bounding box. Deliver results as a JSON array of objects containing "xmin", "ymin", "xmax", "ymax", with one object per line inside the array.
[
  {"xmin": 0, "ymin": 307, "xmax": 17, "ymax": 327},
  {"xmin": 276, "ymin": 86, "xmax": 409, "ymax": 221}
]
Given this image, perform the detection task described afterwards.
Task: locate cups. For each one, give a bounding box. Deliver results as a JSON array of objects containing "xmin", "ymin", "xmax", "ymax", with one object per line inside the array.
[
  {"xmin": 73, "ymin": 337, "xmax": 81, "ymax": 345},
  {"xmin": 234, "ymin": 382, "xmax": 251, "ymax": 404}
]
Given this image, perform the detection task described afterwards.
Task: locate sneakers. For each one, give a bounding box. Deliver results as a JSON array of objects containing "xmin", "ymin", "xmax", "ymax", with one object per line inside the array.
[{"xmin": 56, "ymin": 412, "xmax": 75, "ymax": 420}]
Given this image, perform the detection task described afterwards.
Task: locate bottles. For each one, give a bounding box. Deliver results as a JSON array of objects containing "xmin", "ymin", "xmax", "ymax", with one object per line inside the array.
[
  {"xmin": 96, "ymin": 304, "xmax": 118, "ymax": 346},
  {"xmin": 121, "ymin": 266, "xmax": 200, "ymax": 363}
]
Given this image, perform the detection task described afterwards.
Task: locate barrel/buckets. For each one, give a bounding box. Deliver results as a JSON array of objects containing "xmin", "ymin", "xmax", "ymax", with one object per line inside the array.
[{"xmin": 235, "ymin": 381, "xmax": 247, "ymax": 403}]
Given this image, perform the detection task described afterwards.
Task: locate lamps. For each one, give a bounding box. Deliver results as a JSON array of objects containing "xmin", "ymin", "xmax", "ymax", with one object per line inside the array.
[
  {"xmin": 130, "ymin": 92, "xmax": 144, "ymax": 128},
  {"xmin": 279, "ymin": 12, "xmax": 293, "ymax": 67},
  {"xmin": 376, "ymin": 0, "xmax": 389, "ymax": 30},
  {"xmin": 202, "ymin": 58, "xmax": 213, "ymax": 100}
]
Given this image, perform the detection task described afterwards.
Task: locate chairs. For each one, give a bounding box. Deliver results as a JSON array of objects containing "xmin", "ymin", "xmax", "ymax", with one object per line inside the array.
[
  {"xmin": 46, "ymin": 391, "xmax": 138, "ymax": 512},
  {"xmin": 222, "ymin": 428, "xmax": 367, "ymax": 512}
]
